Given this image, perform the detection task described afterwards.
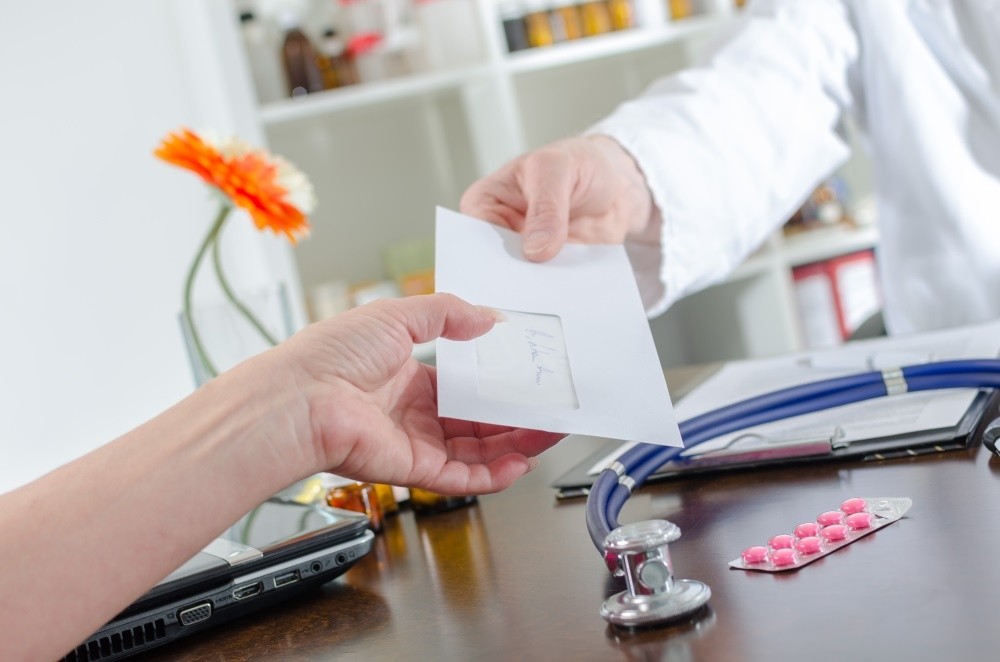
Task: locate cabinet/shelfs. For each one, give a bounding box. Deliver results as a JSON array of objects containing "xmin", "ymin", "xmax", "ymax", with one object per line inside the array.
[{"xmin": 213, "ymin": 1, "xmax": 901, "ymax": 368}]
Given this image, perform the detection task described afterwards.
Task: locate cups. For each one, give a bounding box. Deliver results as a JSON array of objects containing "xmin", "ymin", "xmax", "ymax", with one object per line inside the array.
[{"xmin": 500, "ymin": 0, "xmax": 640, "ymax": 51}]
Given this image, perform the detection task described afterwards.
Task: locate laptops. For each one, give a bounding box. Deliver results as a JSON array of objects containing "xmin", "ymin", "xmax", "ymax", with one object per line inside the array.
[{"xmin": 57, "ymin": 494, "xmax": 376, "ymax": 662}]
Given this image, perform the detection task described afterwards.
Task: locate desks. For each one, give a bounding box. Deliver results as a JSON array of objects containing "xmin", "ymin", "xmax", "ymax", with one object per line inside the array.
[{"xmin": 124, "ymin": 319, "xmax": 999, "ymax": 661}]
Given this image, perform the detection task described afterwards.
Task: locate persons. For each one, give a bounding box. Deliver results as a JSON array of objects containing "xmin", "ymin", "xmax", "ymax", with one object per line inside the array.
[
  {"xmin": 448, "ymin": 3, "xmax": 1000, "ymax": 339},
  {"xmin": 0, "ymin": 291, "xmax": 561, "ymax": 662}
]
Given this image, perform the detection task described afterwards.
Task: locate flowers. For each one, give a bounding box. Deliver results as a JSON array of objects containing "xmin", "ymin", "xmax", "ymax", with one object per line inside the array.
[{"xmin": 150, "ymin": 127, "xmax": 322, "ymax": 373}]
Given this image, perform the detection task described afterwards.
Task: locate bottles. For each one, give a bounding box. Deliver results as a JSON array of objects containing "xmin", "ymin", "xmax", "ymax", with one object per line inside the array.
[
  {"xmin": 320, "ymin": 469, "xmax": 384, "ymax": 534},
  {"xmin": 279, "ymin": 8, "xmax": 359, "ymax": 100}
]
